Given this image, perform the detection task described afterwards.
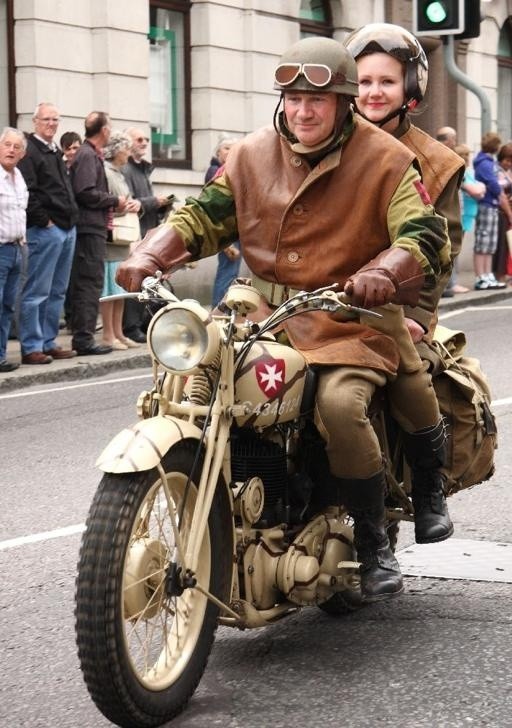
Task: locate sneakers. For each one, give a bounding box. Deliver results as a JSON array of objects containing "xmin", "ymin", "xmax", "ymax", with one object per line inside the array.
[{"xmin": 443, "ymin": 273, "xmax": 508, "ymax": 296}]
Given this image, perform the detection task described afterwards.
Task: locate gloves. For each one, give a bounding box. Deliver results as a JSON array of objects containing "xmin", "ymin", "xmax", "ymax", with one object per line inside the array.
[
  {"xmin": 115, "ymin": 224, "xmax": 192, "ymax": 291},
  {"xmin": 344, "ymin": 249, "xmax": 425, "ymax": 306}
]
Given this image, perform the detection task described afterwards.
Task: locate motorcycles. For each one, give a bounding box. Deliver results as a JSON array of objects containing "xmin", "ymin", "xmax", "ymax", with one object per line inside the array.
[{"xmin": 73, "ymin": 270, "xmax": 462, "ymax": 728}]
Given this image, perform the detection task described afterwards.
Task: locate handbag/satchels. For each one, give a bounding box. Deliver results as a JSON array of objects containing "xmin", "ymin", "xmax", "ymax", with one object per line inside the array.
[
  {"xmin": 104, "ymin": 209, "xmax": 142, "ymax": 246},
  {"xmin": 434, "ymin": 354, "xmax": 496, "ymax": 496}
]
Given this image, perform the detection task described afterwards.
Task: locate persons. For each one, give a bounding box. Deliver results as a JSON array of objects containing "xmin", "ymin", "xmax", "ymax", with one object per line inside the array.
[
  {"xmin": 60, "ymin": 111, "xmax": 127, "ymax": 356},
  {"xmin": 103, "ymin": 130, "xmax": 141, "ymax": 349},
  {"xmin": 0, "ymin": 127, "xmax": 29, "ymax": 373},
  {"xmin": 16, "ymin": 104, "xmax": 78, "ymax": 366},
  {"xmin": 442, "ymin": 120, "xmax": 510, "ymax": 299},
  {"xmin": 205, "ymin": 135, "xmax": 247, "ymax": 308},
  {"xmin": 347, "ymin": 22, "xmax": 463, "ymax": 541},
  {"xmin": 120, "ymin": 123, "xmax": 175, "ymax": 339},
  {"xmin": 115, "ymin": 39, "xmax": 460, "ymax": 600}
]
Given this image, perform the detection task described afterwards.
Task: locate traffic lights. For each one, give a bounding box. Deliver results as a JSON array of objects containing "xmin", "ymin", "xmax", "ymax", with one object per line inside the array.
[{"xmin": 415, "ymin": 0, "xmax": 464, "ymax": 35}]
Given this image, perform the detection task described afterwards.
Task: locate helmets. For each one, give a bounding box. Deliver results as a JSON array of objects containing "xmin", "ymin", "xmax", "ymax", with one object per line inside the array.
[
  {"xmin": 344, "ymin": 22, "xmax": 430, "ymax": 109},
  {"xmin": 275, "ymin": 36, "xmax": 360, "ymax": 98}
]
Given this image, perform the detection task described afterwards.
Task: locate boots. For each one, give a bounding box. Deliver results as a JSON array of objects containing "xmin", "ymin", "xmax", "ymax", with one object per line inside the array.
[
  {"xmin": 335, "ymin": 466, "xmax": 405, "ymax": 604},
  {"xmin": 400, "ymin": 415, "xmax": 454, "ymax": 544}
]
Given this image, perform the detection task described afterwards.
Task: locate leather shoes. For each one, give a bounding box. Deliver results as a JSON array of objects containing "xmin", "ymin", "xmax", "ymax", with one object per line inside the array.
[{"xmin": 0, "ymin": 328, "xmax": 146, "ymax": 371}]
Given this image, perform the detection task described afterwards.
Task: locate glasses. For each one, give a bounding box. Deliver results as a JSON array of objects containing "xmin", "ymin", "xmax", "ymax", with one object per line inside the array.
[{"xmin": 275, "ymin": 63, "xmax": 333, "ymax": 88}]
